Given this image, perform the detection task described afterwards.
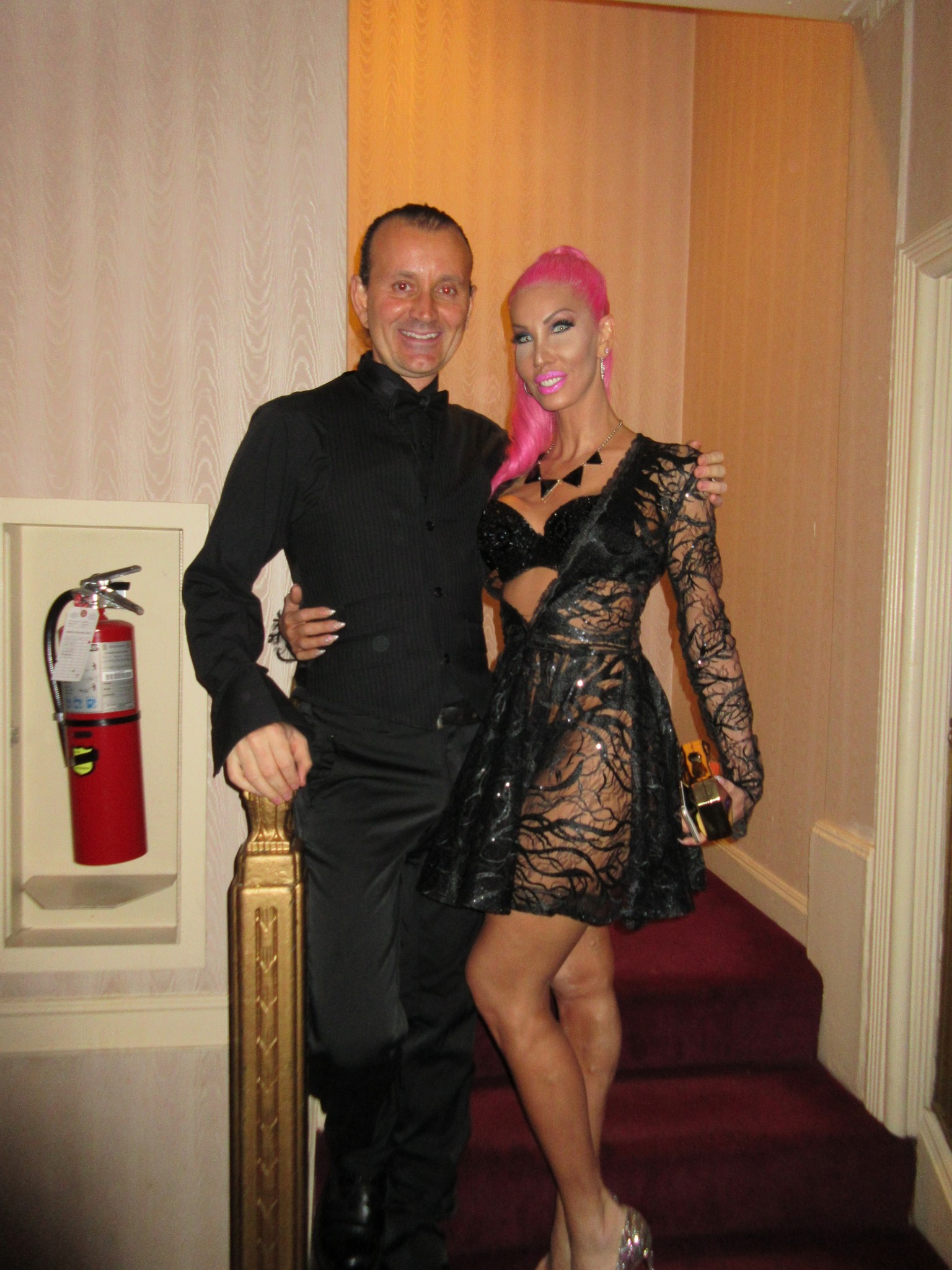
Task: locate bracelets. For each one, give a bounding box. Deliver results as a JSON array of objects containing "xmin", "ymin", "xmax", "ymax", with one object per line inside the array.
[{"xmin": 269, "ymin": 611, "xmax": 300, "ymax": 667}]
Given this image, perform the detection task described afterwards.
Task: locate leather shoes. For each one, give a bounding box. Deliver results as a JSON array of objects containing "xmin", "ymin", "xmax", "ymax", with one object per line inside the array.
[{"xmin": 319, "ymin": 1119, "xmax": 451, "ymax": 1270}]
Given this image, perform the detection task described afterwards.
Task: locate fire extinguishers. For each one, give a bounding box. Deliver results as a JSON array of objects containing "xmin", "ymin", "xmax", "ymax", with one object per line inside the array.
[{"xmin": 42, "ymin": 563, "xmax": 149, "ymax": 866}]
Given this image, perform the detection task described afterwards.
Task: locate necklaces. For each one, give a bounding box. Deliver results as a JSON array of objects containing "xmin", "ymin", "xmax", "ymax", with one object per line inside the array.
[{"xmin": 522, "ymin": 419, "xmax": 623, "ymax": 501}]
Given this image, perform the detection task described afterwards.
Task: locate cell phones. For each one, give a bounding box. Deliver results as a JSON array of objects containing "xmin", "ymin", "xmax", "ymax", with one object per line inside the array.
[{"xmin": 679, "ymin": 779, "xmax": 701, "ymax": 845}]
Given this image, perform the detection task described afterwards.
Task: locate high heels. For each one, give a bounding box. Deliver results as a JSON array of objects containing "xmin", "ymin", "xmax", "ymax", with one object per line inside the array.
[{"xmin": 537, "ymin": 1205, "xmax": 656, "ymax": 1270}]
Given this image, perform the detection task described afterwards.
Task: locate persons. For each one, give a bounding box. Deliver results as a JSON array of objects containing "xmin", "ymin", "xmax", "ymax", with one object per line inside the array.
[
  {"xmin": 177, "ymin": 199, "xmax": 732, "ymax": 1267},
  {"xmin": 268, "ymin": 245, "xmax": 768, "ymax": 1270}
]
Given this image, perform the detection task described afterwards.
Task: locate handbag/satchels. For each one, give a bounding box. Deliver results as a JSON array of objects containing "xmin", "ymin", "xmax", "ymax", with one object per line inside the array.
[{"xmin": 680, "ymin": 739, "xmax": 736, "ymax": 843}]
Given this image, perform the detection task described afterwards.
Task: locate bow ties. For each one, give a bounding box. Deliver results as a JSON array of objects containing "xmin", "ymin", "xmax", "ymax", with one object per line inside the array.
[{"xmin": 388, "ymin": 389, "xmax": 450, "ymax": 426}]
